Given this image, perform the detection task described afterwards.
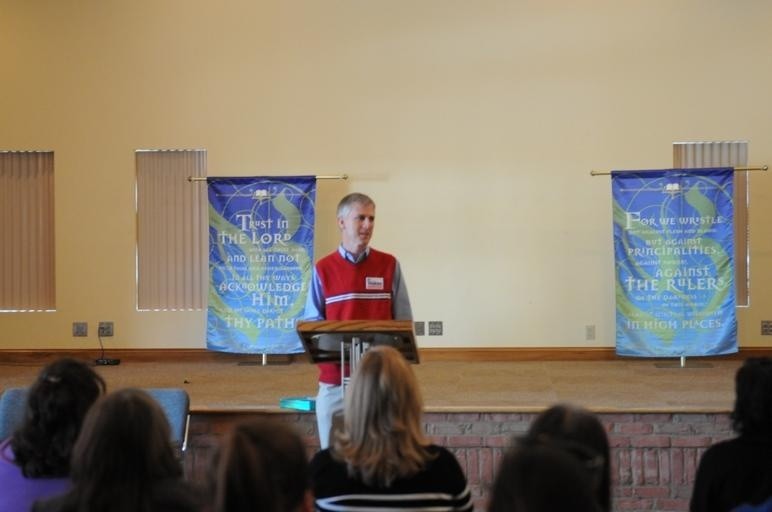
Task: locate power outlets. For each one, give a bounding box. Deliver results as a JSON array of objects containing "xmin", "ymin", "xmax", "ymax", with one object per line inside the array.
[{"xmin": 97, "ymin": 321, "xmax": 113, "ymax": 336}]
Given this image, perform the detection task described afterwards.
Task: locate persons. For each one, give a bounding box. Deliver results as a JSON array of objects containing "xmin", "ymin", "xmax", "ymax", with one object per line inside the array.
[
  {"xmin": 487, "ymin": 404, "xmax": 611, "ymax": 512},
  {"xmin": 305, "ymin": 192, "xmax": 414, "ymax": 451},
  {"xmin": 309, "ymin": 346, "xmax": 474, "ymax": 512},
  {"xmin": 198, "ymin": 416, "xmax": 314, "ymax": 510},
  {"xmin": 1, "ymin": 356, "xmax": 107, "ymax": 510},
  {"xmin": 498, "ymin": 433, "xmax": 614, "ymax": 509},
  {"xmin": 32, "ymin": 387, "xmax": 211, "ymax": 512},
  {"xmin": 687, "ymin": 356, "xmax": 771, "ymax": 511}
]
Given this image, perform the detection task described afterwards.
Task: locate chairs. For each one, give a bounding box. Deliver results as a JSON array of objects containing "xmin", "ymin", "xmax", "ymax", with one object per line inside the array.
[
  {"xmin": 0, "ymin": 386, "xmax": 32, "ymax": 447},
  {"xmin": 132, "ymin": 388, "xmax": 192, "ymax": 464}
]
